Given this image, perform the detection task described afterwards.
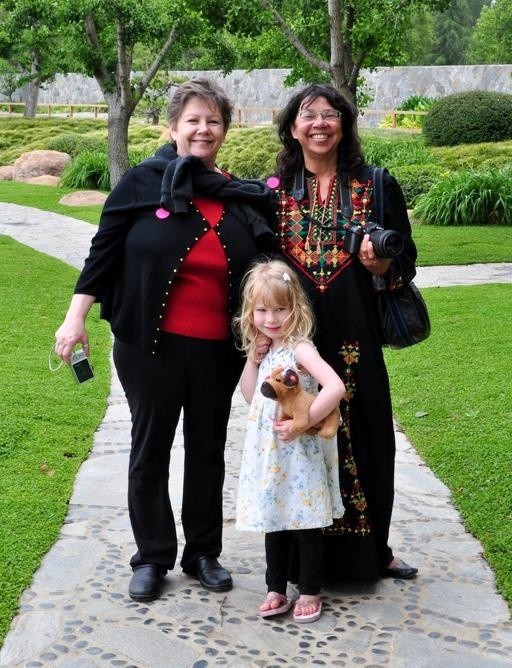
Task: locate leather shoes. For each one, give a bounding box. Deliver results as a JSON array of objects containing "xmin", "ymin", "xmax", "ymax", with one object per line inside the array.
[
  {"xmin": 128, "ymin": 559, "xmax": 165, "ymax": 602},
  {"xmin": 181, "ymin": 548, "xmax": 233, "ymax": 593},
  {"xmin": 386, "ymin": 556, "xmax": 418, "ymax": 579}
]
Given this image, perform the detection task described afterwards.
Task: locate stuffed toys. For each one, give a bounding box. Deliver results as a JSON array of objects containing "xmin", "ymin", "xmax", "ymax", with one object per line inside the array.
[{"xmin": 262, "ymin": 366, "xmax": 342, "ymax": 439}]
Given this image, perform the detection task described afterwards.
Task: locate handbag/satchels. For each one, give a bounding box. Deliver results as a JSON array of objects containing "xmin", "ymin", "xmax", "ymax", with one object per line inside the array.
[{"xmin": 365, "ymin": 165, "xmax": 432, "ymax": 351}]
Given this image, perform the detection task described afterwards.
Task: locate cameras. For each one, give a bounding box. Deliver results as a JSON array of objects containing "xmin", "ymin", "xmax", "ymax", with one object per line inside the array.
[
  {"xmin": 69, "ymin": 349, "xmax": 95, "ymax": 386},
  {"xmin": 343, "ymin": 224, "xmax": 405, "ymax": 259}
]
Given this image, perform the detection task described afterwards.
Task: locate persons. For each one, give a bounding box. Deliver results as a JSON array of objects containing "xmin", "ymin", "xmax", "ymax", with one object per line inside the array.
[
  {"xmin": 52, "ymin": 75, "xmax": 286, "ymax": 604},
  {"xmin": 228, "ymin": 254, "xmax": 349, "ymax": 625},
  {"xmin": 262, "ymin": 84, "xmax": 421, "ymax": 596}
]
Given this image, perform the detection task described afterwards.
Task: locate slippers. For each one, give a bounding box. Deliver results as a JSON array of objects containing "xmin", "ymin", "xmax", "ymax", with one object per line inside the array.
[
  {"xmin": 258, "ymin": 592, "xmax": 293, "ymax": 619},
  {"xmin": 292, "ymin": 592, "xmax": 322, "ymax": 623}
]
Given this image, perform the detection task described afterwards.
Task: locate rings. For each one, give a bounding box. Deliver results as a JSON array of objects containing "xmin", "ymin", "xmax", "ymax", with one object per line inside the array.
[
  {"xmin": 58, "ymin": 345, "xmax": 65, "ymax": 349},
  {"xmin": 359, "ymin": 256, "xmax": 368, "ymax": 261}
]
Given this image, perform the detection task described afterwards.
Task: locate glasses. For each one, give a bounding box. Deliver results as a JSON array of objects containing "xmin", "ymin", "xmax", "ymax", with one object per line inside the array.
[{"xmin": 299, "ymin": 108, "xmax": 343, "ymax": 122}]
